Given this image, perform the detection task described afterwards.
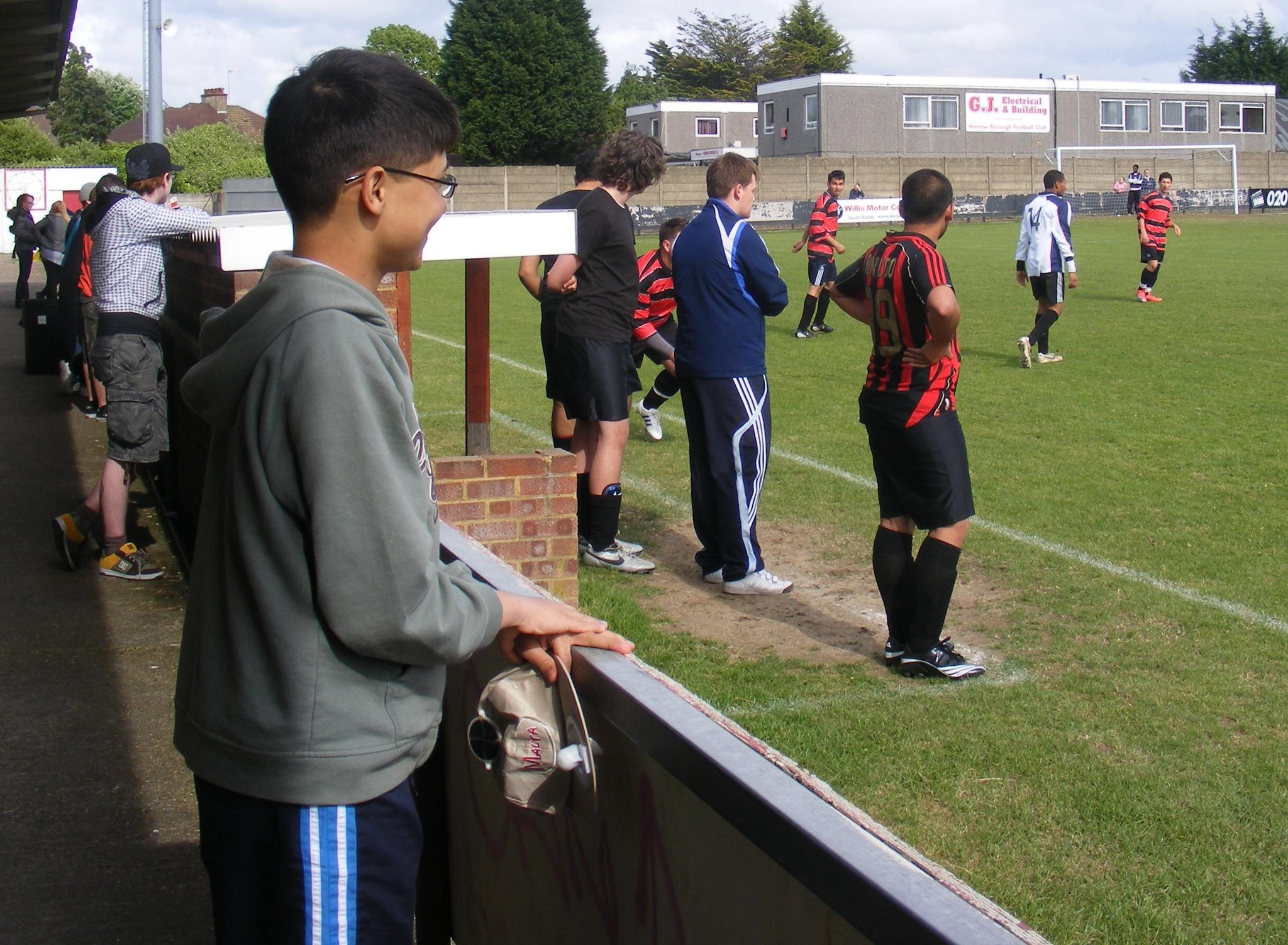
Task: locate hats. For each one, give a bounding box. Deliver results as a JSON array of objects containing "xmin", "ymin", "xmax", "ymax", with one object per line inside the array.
[
  {"xmin": 475, "ymin": 652, "xmax": 598, "ymax": 818},
  {"xmin": 79, "ymin": 182, "xmax": 97, "ymax": 201},
  {"xmin": 126, "ymin": 143, "xmax": 185, "ymax": 181}
]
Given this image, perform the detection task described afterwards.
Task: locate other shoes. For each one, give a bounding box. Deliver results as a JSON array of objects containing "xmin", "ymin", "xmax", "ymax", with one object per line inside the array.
[
  {"xmin": 97, "ymin": 409, "xmax": 108, "ymax": 422},
  {"xmin": 86, "ymin": 406, "xmax": 96, "ymax": 418}
]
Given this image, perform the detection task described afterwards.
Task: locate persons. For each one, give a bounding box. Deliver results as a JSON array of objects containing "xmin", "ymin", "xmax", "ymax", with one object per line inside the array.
[
  {"xmin": 50, "ymin": 143, "xmax": 214, "ymax": 581},
  {"xmin": 637, "ymin": 217, "xmax": 690, "ymax": 442},
  {"xmin": 1135, "ymin": 172, "xmax": 1182, "ymax": 303},
  {"xmin": 848, "ymin": 183, "xmax": 864, "ymax": 200},
  {"xmin": 1140, "ymin": 169, "xmax": 1156, "ymax": 200},
  {"xmin": 1016, "ymin": 169, "xmax": 1078, "ymax": 370},
  {"xmin": 827, "ymin": 168, "xmax": 987, "ymax": 680},
  {"xmin": 545, "ymin": 128, "xmax": 656, "ymax": 575},
  {"xmin": 171, "ymin": 44, "xmax": 639, "ymax": 945},
  {"xmin": 518, "ymin": 143, "xmax": 610, "ymax": 457},
  {"xmin": 1113, "ymin": 177, "xmax": 1128, "ymax": 217},
  {"xmin": 6, "ymin": 194, "xmax": 37, "ymax": 308},
  {"xmin": 32, "ymin": 172, "xmax": 126, "ymax": 421},
  {"xmin": 791, "ymin": 170, "xmax": 846, "ymax": 338},
  {"xmin": 1127, "ymin": 165, "xmax": 1143, "ymax": 217},
  {"xmin": 672, "ymin": 152, "xmax": 795, "ymax": 596}
]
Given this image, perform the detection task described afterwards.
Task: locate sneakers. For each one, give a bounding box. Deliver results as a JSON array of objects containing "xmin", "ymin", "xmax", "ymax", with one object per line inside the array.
[
  {"xmin": 100, "ymin": 540, "xmax": 164, "ymax": 580},
  {"xmin": 60, "ymin": 361, "xmax": 76, "ymax": 394},
  {"xmin": 795, "ymin": 329, "xmax": 818, "ymax": 339},
  {"xmin": 900, "ymin": 638, "xmax": 986, "ymax": 680},
  {"xmin": 884, "ymin": 635, "xmax": 904, "ymax": 665},
  {"xmin": 1145, "ymin": 292, "xmax": 1162, "ymax": 302},
  {"xmin": 1136, "ymin": 289, "xmax": 1147, "ymax": 302},
  {"xmin": 1037, "ymin": 353, "xmax": 1063, "ymax": 363},
  {"xmin": 580, "ymin": 539, "xmax": 644, "ymax": 556},
  {"xmin": 724, "ymin": 569, "xmax": 794, "ymax": 595},
  {"xmin": 704, "ymin": 568, "xmax": 724, "ymax": 583},
  {"xmin": 810, "ymin": 322, "xmax": 834, "ymax": 332},
  {"xmin": 635, "ymin": 399, "xmax": 663, "ymax": 441},
  {"xmin": 583, "ymin": 543, "xmax": 656, "ymax": 574},
  {"xmin": 1017, "ymin": 336, "xmax": 1032, "ymax": 368},
  {"xmin": 54, "ymin": 513, "xmax": 94, "ymax": 573}
]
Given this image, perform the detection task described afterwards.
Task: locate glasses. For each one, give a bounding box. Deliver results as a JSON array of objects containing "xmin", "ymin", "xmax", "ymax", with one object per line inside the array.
[
  {"xmin": 170, "ymin": 173, "xmax": 176, "ymax": 178},
  {"xmin": 345, "ymin": 167, "xmax": 457, "ymax": 198}
]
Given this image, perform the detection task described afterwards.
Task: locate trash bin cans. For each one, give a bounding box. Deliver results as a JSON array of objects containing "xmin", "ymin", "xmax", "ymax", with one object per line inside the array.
[{"xmin": 22, "ymin": 299, "xmax": 76, "ymax": 376}]
